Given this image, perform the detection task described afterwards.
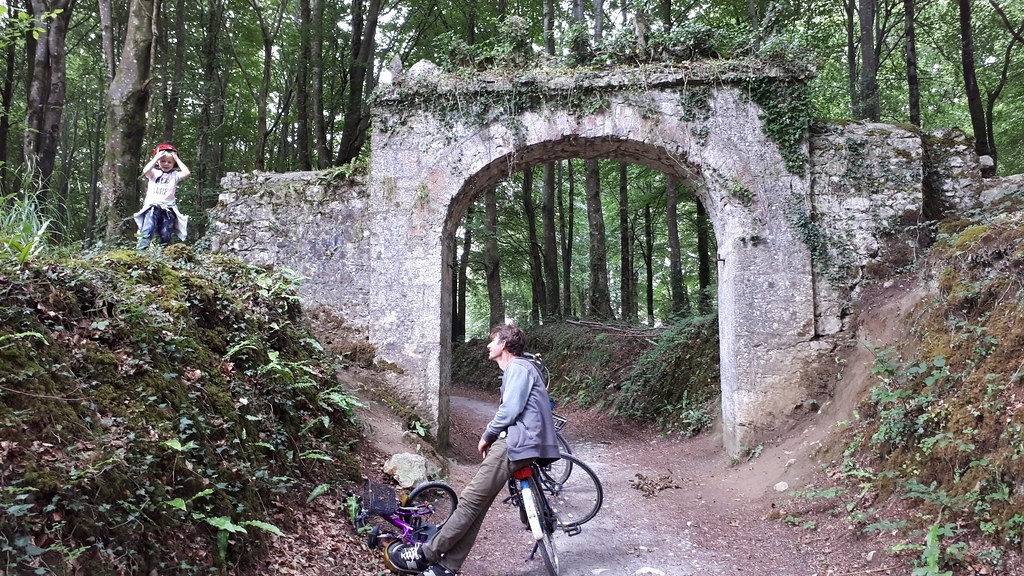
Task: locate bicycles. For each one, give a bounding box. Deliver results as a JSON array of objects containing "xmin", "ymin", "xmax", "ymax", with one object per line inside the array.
[
  {"xmin": 493, "ymin": 352, "xmax": 602, "ymax": 576},
  {"xmin": 353, "ymin": 478, "xmax": 457, "ymax": 574}
]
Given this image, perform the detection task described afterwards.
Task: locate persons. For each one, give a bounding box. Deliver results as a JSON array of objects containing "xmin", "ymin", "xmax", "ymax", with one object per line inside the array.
[
  {"xmin": 389, "ymin": 324, "xmax": 561, "ymax": 576},
  {"xmin": 133, "ymin": 143, "xmax": 191, "ymax": 250}
]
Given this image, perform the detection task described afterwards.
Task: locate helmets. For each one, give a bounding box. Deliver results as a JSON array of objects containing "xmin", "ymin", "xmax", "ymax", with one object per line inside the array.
[{"xmin": 153, "ymin": 144, "xmax": 176, "ymax": 156}]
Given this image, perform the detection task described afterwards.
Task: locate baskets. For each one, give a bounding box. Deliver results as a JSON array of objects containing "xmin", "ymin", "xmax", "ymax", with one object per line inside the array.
[
  {"xmin": 368, "ymin": 483, "xmax": 396, "ymax": 516},
  {"xmin": 552, "ymin": 414, "xmax": 566, "ymax": 432}
]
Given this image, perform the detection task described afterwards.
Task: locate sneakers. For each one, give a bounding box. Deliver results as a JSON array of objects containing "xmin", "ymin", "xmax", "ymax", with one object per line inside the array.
[
  {"xmin": 414, "ymin": 564, "xmax": 458, "ymax": 576},
  {"xmin": 391, "ymin": 543, "xmax": 434, "ymax": 576}
]
